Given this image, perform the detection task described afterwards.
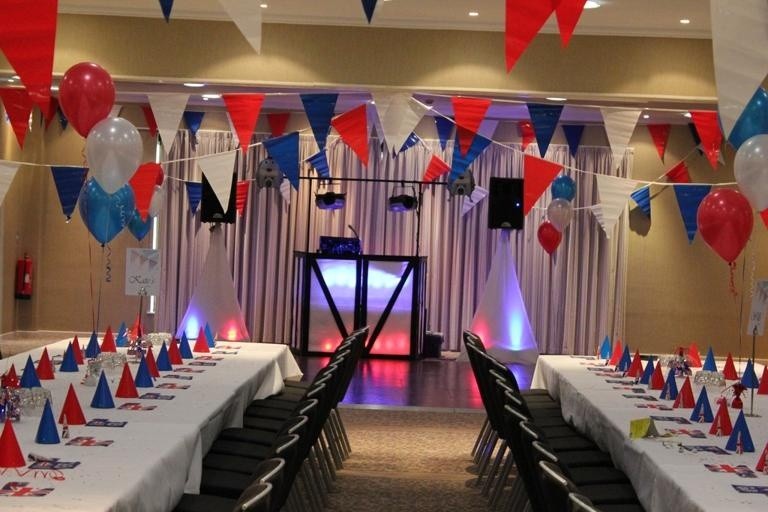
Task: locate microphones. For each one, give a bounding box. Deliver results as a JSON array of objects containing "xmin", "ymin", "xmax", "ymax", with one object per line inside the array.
[{"xmin": 347, "ymin": 224, "xmax": 359, "ymax": 238}]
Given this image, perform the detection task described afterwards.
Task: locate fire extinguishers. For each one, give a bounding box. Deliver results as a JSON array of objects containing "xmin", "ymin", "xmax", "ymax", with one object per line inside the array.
[{"xmin": 15, "ymin": 253, "xmax": 32, "ymax": 299}]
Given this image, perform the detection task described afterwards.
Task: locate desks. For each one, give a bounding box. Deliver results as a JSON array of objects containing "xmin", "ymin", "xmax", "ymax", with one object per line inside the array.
[
  {"xmin": 531, "ymin": 353, "xmax": 768, "ymax": 511},
  {"xmin": 0, "ymin": 334, "xmax": 303, "ymax": 511}
]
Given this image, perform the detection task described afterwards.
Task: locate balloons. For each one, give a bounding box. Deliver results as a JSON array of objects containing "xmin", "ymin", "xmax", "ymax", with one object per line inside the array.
[
  {"xmin": 552, "ymin": 176, "xmax": 579, "ymax": 201},
  {"xmin": 732, "ymin": 88, "xmax": 768, "ymax": 144},
  {"xmin": 547, "ymin": 196, "xmax": 572, "ymax": 231},
  {"xmin": 77, "ymin": 178, "xmax": 153, "ymax": 248},
  {"xmin": 732, "ymin": 134, "xmax": 767, "ymax": 214},
  {"xmin": 58, "ymin": 61, "xmax": 115, "ymax": 140},
  {"xmin": 516, "ymin": 120, "xmax": 536, "ymax": 137},
  {"xmin": 696, "ymin": 187, "xmax": 755, "ymax": 266},
  {"xmin": 536, "ymin": 220, "xmax": 562, "ymax": 255},
  {"xmin": 86, "ymin": 117, "xmax": 144, "ymax": 194}
]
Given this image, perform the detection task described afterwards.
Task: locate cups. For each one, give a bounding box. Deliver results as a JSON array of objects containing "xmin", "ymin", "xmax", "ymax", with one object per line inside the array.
[
  {"xmin": 88, "ymin": 352, "xmax": 127, "ymax": 383},
  {"xmin": 17, "ymin": 387, "xmax": 52, "ymax": 417},
  {"xmin": 694, "ymin": 370, "xmax": 725, "ymax": 387}
]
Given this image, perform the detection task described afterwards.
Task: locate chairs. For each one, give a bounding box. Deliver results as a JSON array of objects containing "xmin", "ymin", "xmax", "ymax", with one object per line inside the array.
[
  {"xmin": 463, "ymin": 330, "xmax": 646, "ymax": 511},
  {"xmin": 169, "ymin": 324, "xmax": 369, "ymax": 511}
]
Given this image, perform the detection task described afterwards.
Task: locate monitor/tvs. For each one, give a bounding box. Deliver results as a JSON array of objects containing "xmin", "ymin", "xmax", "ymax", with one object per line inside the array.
[{"xmin": 319, "ymin": 235, "xmax": 360, "ymax": 256}]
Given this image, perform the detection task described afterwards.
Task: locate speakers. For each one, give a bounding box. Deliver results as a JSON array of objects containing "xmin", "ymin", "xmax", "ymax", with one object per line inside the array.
[
  {"xmin": 200, "ymin": 172, "xmax": 237, "ymax": 225},
  {"xmin": 487, "ymin": 175, "xmax": 524, "ymax": 231}
]
